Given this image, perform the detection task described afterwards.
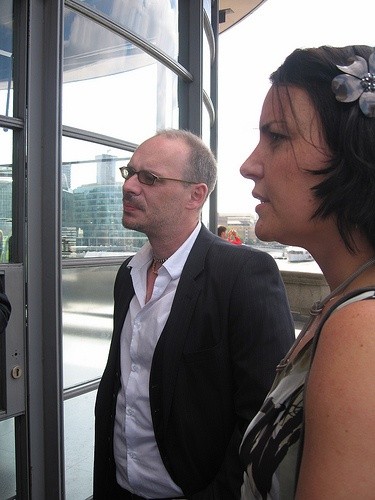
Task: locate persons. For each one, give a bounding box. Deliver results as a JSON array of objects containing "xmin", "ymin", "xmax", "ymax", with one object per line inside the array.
[
  {"xmin": 93, "ymin": 129, "xmax": 296, "ymax": 500},
  {"xmin": 238, "ymin": 45, "xmax": 375, "ymax": 500},
  {"xmin": 218, "ymin": 226, "xmax": 241, "ymax": 245}
]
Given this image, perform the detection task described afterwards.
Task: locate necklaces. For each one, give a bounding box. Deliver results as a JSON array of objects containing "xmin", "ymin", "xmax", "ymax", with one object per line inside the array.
[
  {"xmin": 153, "ymin": 257, "xmax": 168, "ymax": 273},
  {"xmin": 273, "ymin": 256, "xmax": 375, "ymax": 382}
]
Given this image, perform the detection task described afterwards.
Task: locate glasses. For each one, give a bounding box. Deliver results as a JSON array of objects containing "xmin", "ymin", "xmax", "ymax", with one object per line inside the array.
[{"xmin": 119, "ymin": 167, "xmax": 198, "ymax": 186}]
